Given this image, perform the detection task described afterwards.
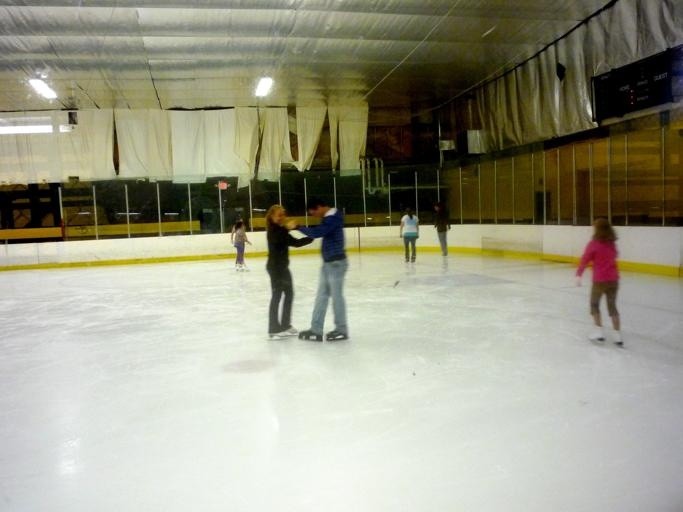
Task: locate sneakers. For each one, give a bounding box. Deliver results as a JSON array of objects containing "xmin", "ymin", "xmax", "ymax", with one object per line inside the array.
[
  {"xmin": 589, "ymin": 331, "xmax": 604, "ymax": 341},
  {"xmin": 298, "ymin": 330, "xmax": 322, "ymax": 336},
  {"xmin": 327, "ymin": 331, "xmax": 346, "ymax": 337},
  {"xmin": 235, "ymin": 264, "xmax": 247, "ymax": 271},
  {"xmin": 268, "ymin": 326, "xmax": 297, "ymax": 337},
  {"xmin": 612, "ymin": 333, "xmax": 622, "ymax": 344}
]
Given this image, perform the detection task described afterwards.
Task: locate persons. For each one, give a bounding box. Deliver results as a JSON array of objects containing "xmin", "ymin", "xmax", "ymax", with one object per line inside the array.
[
  {"xmin": 434, "ymin": 202, "xmax": 451, "ymax": 256},
  {"xmin": 264, "ymin": 204, "xmax": 314, "ymax": 336},
  {"xmin": 575, "ymin": 218, "xmax": 624, "ymax": 345},
  {"xmin": 286, "ymin": 197, "xmax": 350, "ymax": 337},
  {"xmin": 232, "ymin": 218, "xmax": 246, "ymax": 233},
  {"xmin": 400, "ymin": 207, "xmax": 420, "ymax": 262},
  {"xmin": 231, "ymin": 221, "xmax": 252, "ymax": 270}
]
